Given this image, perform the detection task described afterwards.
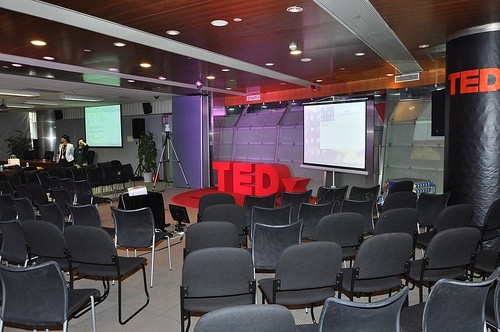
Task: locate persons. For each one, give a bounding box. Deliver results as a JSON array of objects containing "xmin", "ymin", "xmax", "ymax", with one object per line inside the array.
[
  {"xmin": 55, "ymin": 135, "xmax": 75, "ymax": 168},
  {"xmin": 74, "ymin": 138, "xmax": 90, "ymax": 167}
]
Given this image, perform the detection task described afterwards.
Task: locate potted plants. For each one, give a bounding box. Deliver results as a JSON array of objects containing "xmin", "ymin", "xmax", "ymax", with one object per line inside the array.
[{"xmin": 136, "ymin": 129, "xmax": 155, "ymax": 183}]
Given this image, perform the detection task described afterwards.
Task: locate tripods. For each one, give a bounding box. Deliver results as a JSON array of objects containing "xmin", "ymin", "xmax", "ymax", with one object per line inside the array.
[{"xmin": 152, "ymin": 135, "xmax": 189, "ymax": 192}]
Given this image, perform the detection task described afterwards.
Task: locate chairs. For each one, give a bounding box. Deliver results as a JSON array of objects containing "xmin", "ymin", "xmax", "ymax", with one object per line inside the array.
[
  {"xmin": 86, "ymin": 150, "xmax": 95, "ymax": 165},
  {"xmin": 0, "ymin": 160, "xmax": 174, "ymax": 332},
  {"xmin": 181, "ymin": 179, "xmax": 500, "ymax": 332},
  {"xmin": 44, "ymin": 151, "xmax": 54, "ymax": 160}
]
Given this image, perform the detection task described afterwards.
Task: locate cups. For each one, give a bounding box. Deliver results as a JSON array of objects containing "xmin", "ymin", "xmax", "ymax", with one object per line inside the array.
[
  {"xmin": 53, "ymin": 157, "xmax": 56, "ymax": 161},
  {"xmin": 43, "ymin": 159, "xmax": 46, "ymax": 162}
]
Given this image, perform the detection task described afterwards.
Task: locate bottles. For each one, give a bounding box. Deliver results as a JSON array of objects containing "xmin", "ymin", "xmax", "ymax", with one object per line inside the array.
[
  {"xmin": 0, "ymin": 165, "xmax": 3, "ymax": 171},
  {"xmin": 26, "ymin": 162, "xmax": 30, "ymax": 167}
]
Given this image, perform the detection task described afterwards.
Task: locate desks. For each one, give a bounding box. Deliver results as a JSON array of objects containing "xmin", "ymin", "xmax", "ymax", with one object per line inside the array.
[
  {"xmin": 27, "ymin": 161, "xmax": 62, "ymax": 168},
  {"xmin": 118, "ymin": 192, "xmax": 166, "ymax": 232}
]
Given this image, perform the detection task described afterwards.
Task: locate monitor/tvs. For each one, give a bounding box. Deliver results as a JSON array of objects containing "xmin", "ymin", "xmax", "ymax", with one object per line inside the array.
[{"xmin": 169, "ymin": 204, "xmax": 190, "ymax": 227}]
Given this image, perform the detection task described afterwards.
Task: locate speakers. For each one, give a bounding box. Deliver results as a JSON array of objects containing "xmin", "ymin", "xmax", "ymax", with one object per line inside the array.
[
  {"xmin": 142, "ymin": 102, "xmax": 153, "ymax": 115},
  {"xmin": 54, "ymin": 110, "xmax": 63, "ymax": 120}
]
